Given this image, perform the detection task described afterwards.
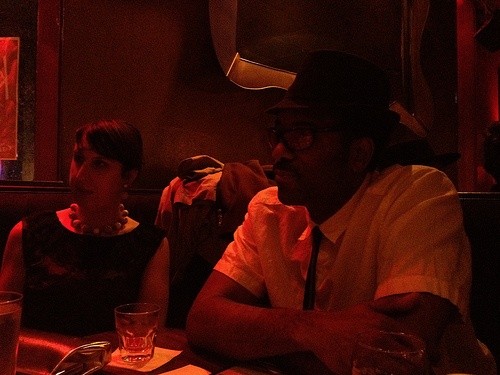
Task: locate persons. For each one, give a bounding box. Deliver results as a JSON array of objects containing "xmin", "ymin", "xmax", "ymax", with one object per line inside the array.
[
  {"xmin": 187, "ymin": 49, "xmax": 498, "ymax": 374},
  {"xmin": 0, "ymin": 117, "xmax": 169, "ymax": 338}
]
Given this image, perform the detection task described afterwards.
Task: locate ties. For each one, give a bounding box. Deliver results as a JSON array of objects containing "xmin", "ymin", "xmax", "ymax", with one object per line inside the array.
[{"xmin": 303, "ymin": 226, "xmax": 324, "ymax": 310}]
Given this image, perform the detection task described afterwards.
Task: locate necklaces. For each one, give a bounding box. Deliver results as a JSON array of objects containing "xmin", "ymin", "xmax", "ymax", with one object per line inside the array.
[{"xmin": 69, "ymin": 202, "xmax": 129, "ymax": 234}]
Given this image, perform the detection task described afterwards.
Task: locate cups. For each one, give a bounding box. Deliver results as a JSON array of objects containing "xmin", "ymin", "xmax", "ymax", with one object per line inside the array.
[
  {"xmin": 351, "ymin": 332, "xmax": 425, "ymax": 375},
  {"xmin": 0, "ymin": 291, "xmax": 23, "ymax": 375},
  {"xmin": 114, "ymin": 303, "xmax": 161, "ymax": 362}
]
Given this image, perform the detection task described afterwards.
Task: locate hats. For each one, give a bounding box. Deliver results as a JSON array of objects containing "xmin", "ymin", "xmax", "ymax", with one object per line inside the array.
[{"xmin": 266, "ymin": 50, "xmax": 390, "ymax": 135}]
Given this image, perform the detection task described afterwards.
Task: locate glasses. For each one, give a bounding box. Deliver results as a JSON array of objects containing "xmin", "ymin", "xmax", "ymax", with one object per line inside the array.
[{"xmin": 268, "ymin": 126, "xmax": 345, "ymax": 152}]
